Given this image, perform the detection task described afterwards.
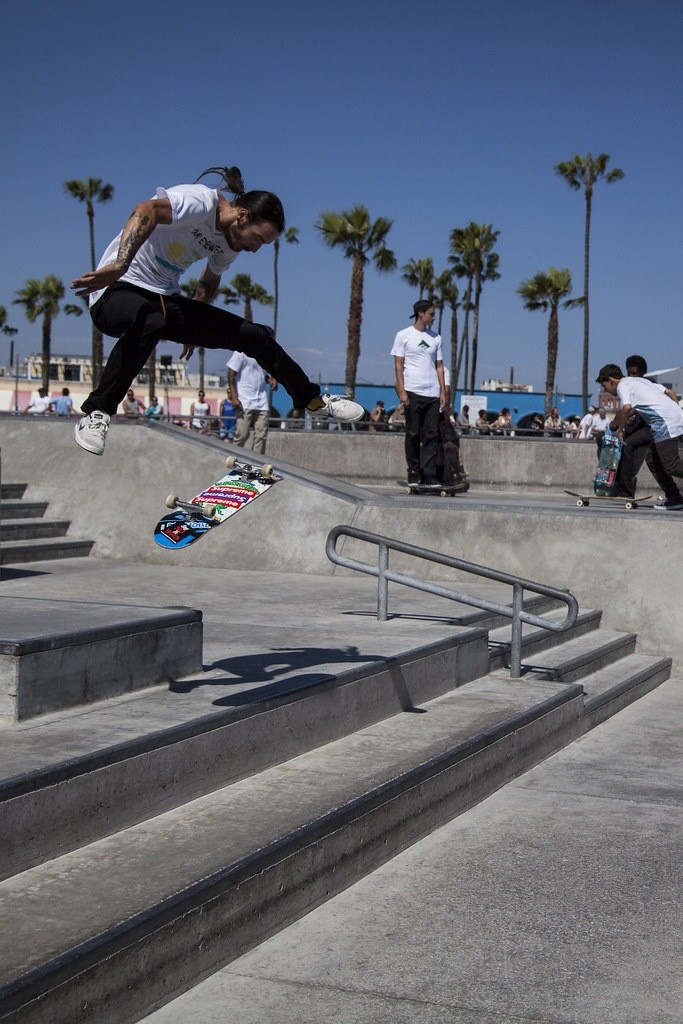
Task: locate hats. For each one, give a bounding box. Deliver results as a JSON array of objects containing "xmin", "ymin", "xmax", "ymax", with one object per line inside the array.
[
  {"xmin": 574, "ymin": 416, "xmax": 581, "ymax": 420},
  {"xmin": 409, "ymin": 300, "xmax": 433, "ymax": 318},
  {"xmin": 588, "ymin": 406, "xmax": 596, "ymax": 413}
]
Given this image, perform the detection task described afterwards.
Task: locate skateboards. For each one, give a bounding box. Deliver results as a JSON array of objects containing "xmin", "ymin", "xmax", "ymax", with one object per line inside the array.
[
  {"xmin": 396, "ymin": 479, "xmax": 470, "ymax": 498},
  {"xmin": 560, "ymin": 489, "xmax": 653, "ymax": 510},
  {"xmin": 593, "ymin": 428, "xmax": 626, "ymax": 495},
  {"xmin": 150, "ymin": 454, "xmax": 285, "ymax": 550}
]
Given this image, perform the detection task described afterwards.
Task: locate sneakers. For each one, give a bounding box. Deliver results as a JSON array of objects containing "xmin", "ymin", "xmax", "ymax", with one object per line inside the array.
[
  {"xmin": 75, "ymin": 409, "xmax": 111, "ymax": 456},
  {"xmin": 304, "ymin": 392, "xmax": 365, "ymax": 424}
]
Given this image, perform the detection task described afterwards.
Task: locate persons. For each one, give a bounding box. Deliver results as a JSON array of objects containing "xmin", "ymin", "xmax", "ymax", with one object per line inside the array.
[
  {"xmin": 70, "ymin": 166, "xmax": 365, "ymax": 457},
  {"xmin": 595, "ymin": 354, "xmax": 683, "ymax": 512},
  {"xmin": 227, "ymin": 348, "xmax": 278, "ymax": 456},
  {"xmin": 23, "ymin": 387, "xmax": 611, "ymax": 445},
  {"xmin": 389, "ymin": 298, "xmax": 451, "ymax": 489}
]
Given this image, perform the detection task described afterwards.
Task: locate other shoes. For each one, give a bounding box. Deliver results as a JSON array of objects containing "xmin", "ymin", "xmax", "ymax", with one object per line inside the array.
[
  {"xmin": 407, "ymin": 473, "xmax": 421, "ymax": 487},
  {"xmin": 653, "ymin": 495, "xmax": 683, "ymax": 510}
]
